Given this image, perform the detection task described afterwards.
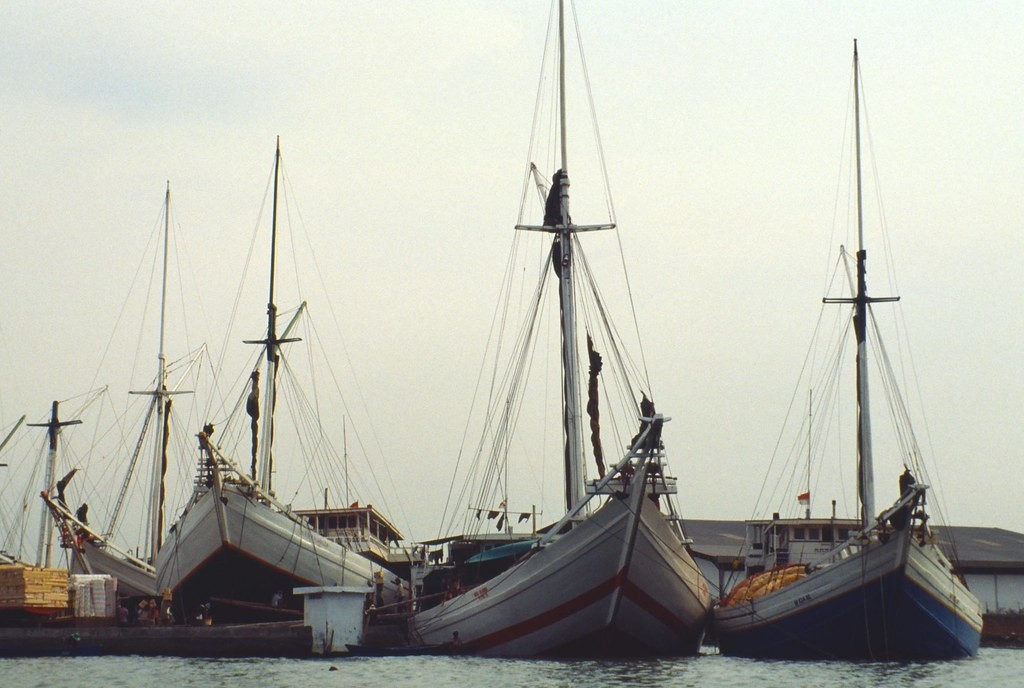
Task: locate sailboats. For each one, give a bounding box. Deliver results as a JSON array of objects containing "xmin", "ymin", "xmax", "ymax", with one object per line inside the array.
[
  {"xmin": 0, "ymin": 180, "xmax": 178, "ymax": 600},
  {"xmin": 152, "ymin": 136, "xmax": 415, "ymax": 615},
  {"xmin": 710, "ymin": 38, "xmax": 985, "ymax": 659},
  {"xmin": 406, "ymin": 1, "xmax": 713, "ymax": 658}
]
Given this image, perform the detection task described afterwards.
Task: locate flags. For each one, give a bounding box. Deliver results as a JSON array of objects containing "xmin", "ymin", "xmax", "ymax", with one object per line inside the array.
[{"xmin": 798, "ymin": 493, "xmax": 809, "ymax": 505}]
